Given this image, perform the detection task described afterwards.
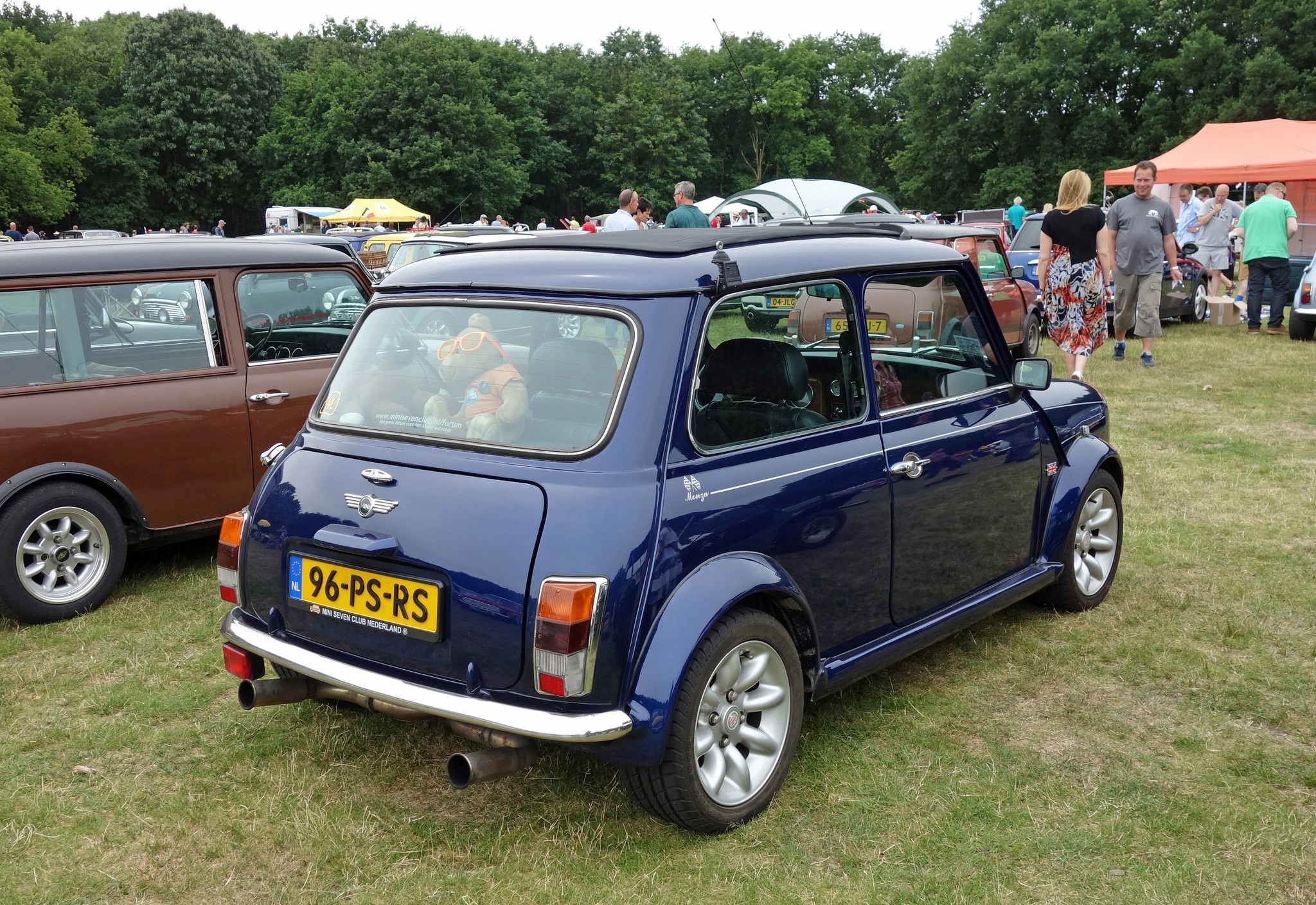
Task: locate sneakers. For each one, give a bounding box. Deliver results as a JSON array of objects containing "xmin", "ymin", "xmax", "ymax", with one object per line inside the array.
[
  {"xmin": 1113, "ymin": 343, "xmax": 1126, "ymax": 361},
  {"xmin": 1139, "ymin": 352, "xmax": 1155, "ymax": 368}
]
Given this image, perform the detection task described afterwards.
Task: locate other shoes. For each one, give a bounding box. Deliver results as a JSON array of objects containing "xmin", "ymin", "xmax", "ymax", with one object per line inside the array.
[
  {"xmin": 1071, "ymin": 371, "xmax": 1083, "ymax": 380},
  {"xmin": 1267, "ymin": 325, "xmax": 1289, "ymax": 335},
  {"xmin": 1240, "ymin": 328, "xmax": 1260, "ymax": 334}
]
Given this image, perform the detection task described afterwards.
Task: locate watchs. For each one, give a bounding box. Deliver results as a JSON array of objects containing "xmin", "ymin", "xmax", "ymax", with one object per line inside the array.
[{"xmin": 1194, "ymin": 226, "xmax": 1199, "ymax": 232}]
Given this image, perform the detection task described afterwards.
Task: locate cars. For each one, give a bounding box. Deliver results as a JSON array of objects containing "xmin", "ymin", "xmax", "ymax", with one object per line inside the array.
[
  {"xmin": 0, "ymin": 234, "xmax": 445, "ymax": 625},
  {"xmin": 130, "ymin": 177, "xmax": 1237, "ymax": 357},
  {"xmin": 1289, "ymin": 249, "xmax": 1316, "ymax": 342},
  {"xmin": 216, "ymin": 235, "xmax": 1126, "ymax": 836}
]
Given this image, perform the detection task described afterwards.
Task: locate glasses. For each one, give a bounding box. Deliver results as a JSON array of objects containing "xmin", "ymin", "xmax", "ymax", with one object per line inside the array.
[
  {"xmin": 673, "ymin": 192, "xmax": 680, "ymax": 197},
  {"xmin": 1179, "ymin": 194, "xmax": 1189, "ymax": 198},
  {"xmin": 627, "ymin": 188, "xmax": 635, "ymax": 203},
  {"xmin": 903, "ymin": 211, "xmax": 906, "ymax": 213},
  {"xmin": 482, "ymin": 218, "xmax": 487, "ymax": 219},
  {"xmin": 438, "ymin": 332, "xmax": 512, "ymax": 364}
]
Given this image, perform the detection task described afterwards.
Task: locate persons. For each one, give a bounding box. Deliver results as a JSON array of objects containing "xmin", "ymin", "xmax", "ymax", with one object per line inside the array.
[
  {"xmin": 132, "ymin": 222, "xmax": 199, "ymax": 234},
  {"xmin": 866, "ymin": 205, "xmax": 961, "ymax": 224},
  {"xmin": 603, "ymin": 181, "xmax": 712, "ymax": 232},
  {"xmin": 1038, "ymin": 169, "xmax": 1113, "ymax": 380},
  {"xmin": 411, "ymin": 216, "xmax": 439, "ymax": 232},
  {"xmin": 1176, "ymin": 183, "xmax": 1299, "ymax": 334},
  {"xmin": 862, "ymin": 210, "xmax": 867, "ymax": 214},
  {"xmin": 471, "ymin": 214, "xmax": 600, "ymax": 233},
  {"xmin": 709, "ymin": 208, "xmax": 755, "ymax": 229},
  {"xmin": 1007, "ymin": 197, "xmax": 1025, "ymax": 235},
  {"xmin": 269, "ymin": 224, "xmax": 304, "ymax": 233},
  {"xmin": 0, "ymin": 222, "xmax": 78, "ymax": 242},
  {"xmin": 1106, "ymin": 160, "xmax": 1183, "ymax": 368},
  {"xmin": 321, "ymin": 220, "xmax": 396, "ymax": 235},
  {"xmin": 215, "ymin": 220, "xmax": 226, "ymax": 236}
]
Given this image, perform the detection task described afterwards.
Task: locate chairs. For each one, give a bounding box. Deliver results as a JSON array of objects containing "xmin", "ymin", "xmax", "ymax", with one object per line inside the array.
[
  {"xmin": 695, "ymin": 337, "xmax": 830, "ymax": 446},
  {"xmin": 525, "ymin": 337, "xmax": 618, "ymax": 423}
]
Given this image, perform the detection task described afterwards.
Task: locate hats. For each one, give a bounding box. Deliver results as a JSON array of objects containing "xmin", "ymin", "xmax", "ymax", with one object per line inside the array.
[
  {"xmin": 480, "ymin": 214, "xmax": 488, "ymax": 218},
  {"xmin": 415, "ymin": 216, "xmax": 421, "ymax": 221},
  {"xmin": 420, "ymin": 216, "xmax": 429, "ymax": 223},
  {"xmin": 219, "ymin": 220, "xmax": 226, "ymax": 224},
  {"xmin": 300, "ymin": 224, "xmax": 304, "ymax": 228},
  {"xmin": 732, "ymin": 212, "xmax": 741, "ymax": 217},
  {"xmin": 53, "ymin": 231, "xmax": 60, "ymax": 234},
  {"xmin": 343, "ymin": 221, "xmax": 348, "ymax": 224},
  {"xmin": 868, "ymin": 205, "xmax": 878, "ymax": 213}
]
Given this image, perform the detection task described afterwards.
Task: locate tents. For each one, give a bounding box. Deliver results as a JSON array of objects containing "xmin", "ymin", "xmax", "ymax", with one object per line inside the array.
[
  {"xmin": 693, "ymin": 196, "xmax": 758, "ymax": 224},
  {"xmin": 319, "ymin": 198, "xmax": 431, "ymax": 234},
  {"xmin": 707, "ymin": 178, "xmax": 902, "ymax": 225},
  {"xmin": 1104, "ymin": 118, "xmax": 1316, "ymax": 258}
]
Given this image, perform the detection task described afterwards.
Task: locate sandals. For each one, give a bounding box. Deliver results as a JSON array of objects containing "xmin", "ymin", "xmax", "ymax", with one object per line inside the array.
[{"xmin": 1225, "ymin": 281, "xmax": 1237, "ymax": 297}]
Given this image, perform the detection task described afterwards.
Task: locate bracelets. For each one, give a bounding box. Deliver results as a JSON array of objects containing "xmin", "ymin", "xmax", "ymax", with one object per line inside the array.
[
  {"xmin": 1170, "ymin": 266, "xmax": 1178, "ymax": 270},
  {"xmin": 1040, "ymin": 288, "xmax": 1045, "ymax": 297},
  {"xmin": 1105, "ymin": 285, "xmax": 1110, "ymax": 287}
]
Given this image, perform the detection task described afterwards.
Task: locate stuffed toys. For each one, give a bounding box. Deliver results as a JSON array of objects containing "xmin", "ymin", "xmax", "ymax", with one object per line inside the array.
[{"xmin": 423, "ymin": 313, "xmax": 529, "ymax": 442}]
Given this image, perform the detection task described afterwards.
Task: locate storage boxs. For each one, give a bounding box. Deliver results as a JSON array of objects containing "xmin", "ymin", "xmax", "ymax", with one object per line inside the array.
[
  {"xmin": 1203, "ymin": 295, "xmax": 1248, "ymax": 326},
  {"xmin": 1166, "ymin": 292, "xmax": 1189, "ymax": 306}
]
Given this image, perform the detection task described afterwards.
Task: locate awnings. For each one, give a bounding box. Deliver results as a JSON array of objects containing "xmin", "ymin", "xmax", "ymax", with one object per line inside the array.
[{"xmin": 293, "ymin": 208, "xmax": 339, "ymax": 219}]
